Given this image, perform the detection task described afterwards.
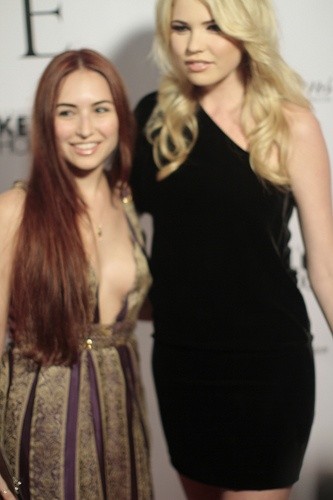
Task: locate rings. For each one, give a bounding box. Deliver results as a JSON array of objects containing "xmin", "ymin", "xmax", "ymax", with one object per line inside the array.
[{"xmin": 0, "ymin": 489, "xmax": 10, "ymax": 497}]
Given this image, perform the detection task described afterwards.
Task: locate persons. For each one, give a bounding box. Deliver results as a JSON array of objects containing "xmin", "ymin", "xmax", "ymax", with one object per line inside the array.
[
  {"xmin": 125, "ymin": 1, "xmax": 333, "ymax": 500},
  {"xmin": 0, "ymin": 46, "xmax": 156, "ymax": 500}
]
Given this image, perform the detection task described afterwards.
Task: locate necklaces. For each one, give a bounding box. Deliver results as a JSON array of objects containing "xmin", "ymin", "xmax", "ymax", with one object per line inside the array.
[{"xmin": 77, "ymin": 171, "xmax": 106, "ymax": 239}]
